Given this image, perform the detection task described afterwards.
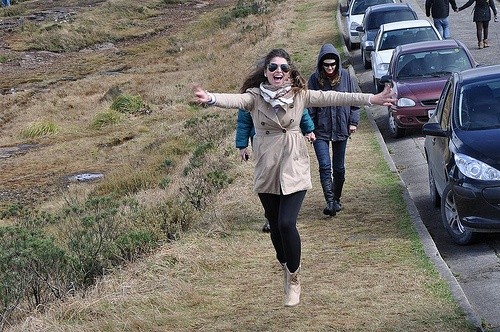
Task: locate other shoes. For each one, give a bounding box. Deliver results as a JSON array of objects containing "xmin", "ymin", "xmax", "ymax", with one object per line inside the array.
[{"xmin": 262, "ymin": 222, "xmax": 269, "ymax": 231}]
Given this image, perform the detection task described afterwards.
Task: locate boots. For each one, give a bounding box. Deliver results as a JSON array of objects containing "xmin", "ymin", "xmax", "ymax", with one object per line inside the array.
[
  {"xmin": 478, "ymin": 40, "xmax": 483, "ymax": 49},
  {"xmin": 333, "ymin": 182, "xmax": 345, "ymax": 211},
  {"xmin": 323, "ymin": 191, "xmax": 336, "ymax": 216},
  {"xmin": 282, "ymin": 262, "xmax": 301, "ymax": 307},
  {"xmin": 484, "ymin": 39, "xmax": 491, "ymax": 47}
]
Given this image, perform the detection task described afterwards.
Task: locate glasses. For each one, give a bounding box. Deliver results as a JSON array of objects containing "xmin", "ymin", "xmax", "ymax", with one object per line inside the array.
[
  {"xmin": 268, "ymin": 63, "xmax": 290, "ymax": 73},
  {"xmin": 323, "ymin": 62, "xmax": 336, "ymax": 67}
]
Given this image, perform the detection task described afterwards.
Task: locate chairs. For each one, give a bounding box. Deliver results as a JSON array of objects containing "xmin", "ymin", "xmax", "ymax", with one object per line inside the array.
[
  {"xmin": 402, "ymin": 55, "xmax": 424, "ymax": 73},
  {"xmin": 416, "ymin": 30, "xmax": 427, "ymax": 41},
  {"xmin": 466, "ymin": 85, "xmax": 500, "ymax": 124}
]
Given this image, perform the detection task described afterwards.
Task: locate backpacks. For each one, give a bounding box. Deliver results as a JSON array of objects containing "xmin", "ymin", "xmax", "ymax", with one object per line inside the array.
[{"xmin": 475, "ymin": 0, "xmax": 488, "ymax": 16}]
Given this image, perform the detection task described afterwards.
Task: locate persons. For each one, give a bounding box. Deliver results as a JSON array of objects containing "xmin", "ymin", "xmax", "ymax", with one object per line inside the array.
[
  {"xmin": 236, "ymin": 107, "xmax": 317, "ymax": 232},
  {"xmin": 425, "ymin": 0, "xmax": 457, "ymax": 39},
  {"xmin": 457, "ymin": 0, "xmax": 497, "ymax": 49},
  {"xmin": 192, "ymin": 48, "xmax": 398, "ymax": 305},
  {"xmin": 308, "ymin": 44, "xmax": 362, "ymax": 218}
]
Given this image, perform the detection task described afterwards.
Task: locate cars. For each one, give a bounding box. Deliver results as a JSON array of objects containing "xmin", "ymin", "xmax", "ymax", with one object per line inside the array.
[
  {"xmin": 341, "ymin": 0, "xmax": 397, "ymax": 49},
  {"xmin": 356, "ymin": 2, "xmax": 421, "ymax": 70},
  {"xmin": 380, "ymin": 37, "xmax": 481, "ymax": 138},
  {"xmin": 421, "ymin": 63, "xmax": 500, "ymax": 247},
  {"xmin": 365, "ymin": 18, "xmax": 444, "ymax": 95}
]
